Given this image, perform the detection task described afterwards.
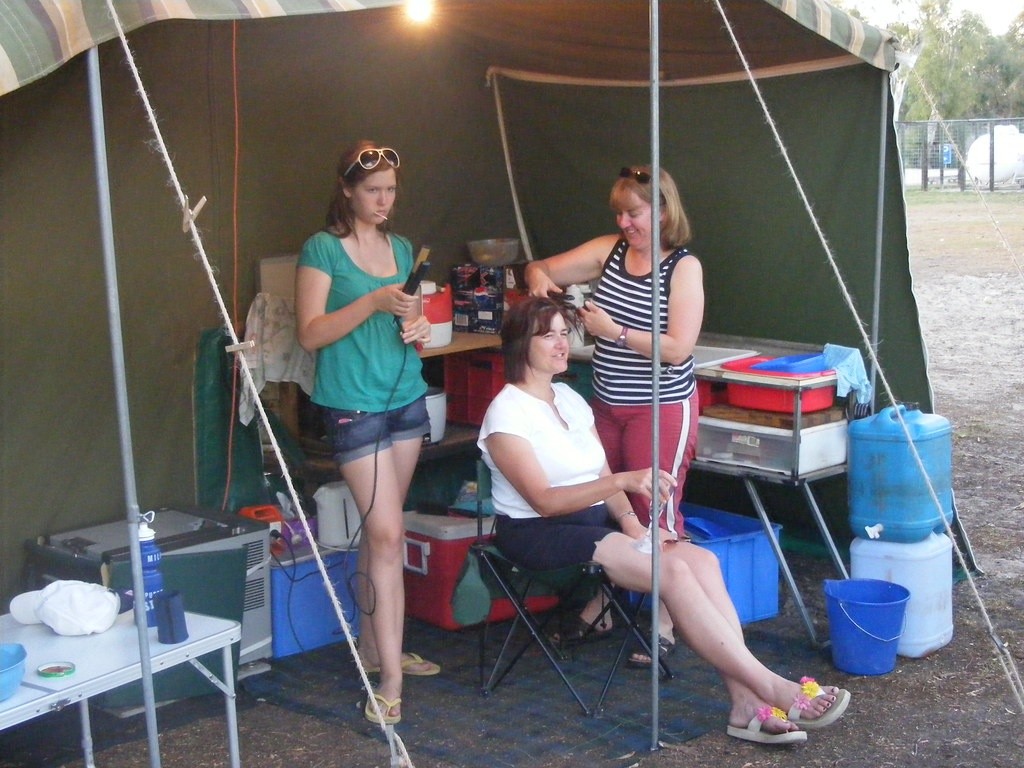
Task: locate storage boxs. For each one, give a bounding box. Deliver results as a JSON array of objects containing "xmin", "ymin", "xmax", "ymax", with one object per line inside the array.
[
  {"xmin": 553, "ymin": 360, "xmax": 595, "ymax": 402},
  {"xmin": 400, "ymin": 509, "xmax": 560, "ymax": 629},
  {"xmin": 627, "ymin": 501, "xmax": 782, "ymax": 627},
  {"xmin": 442, "ymin": 350, "xmax": 512, "ymax": 426},
  {"xmin": 453, "ymin": 261, "xmax": 527, "ymax": 336},
  {"xmin": 268, "ymin": 537, "xmax": 360, "ymax": 659}
]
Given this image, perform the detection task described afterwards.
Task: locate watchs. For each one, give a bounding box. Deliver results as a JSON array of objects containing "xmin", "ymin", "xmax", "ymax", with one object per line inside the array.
[{"xmin": 616, "ymin": 326, "xmax": 628, "ymax": 348}]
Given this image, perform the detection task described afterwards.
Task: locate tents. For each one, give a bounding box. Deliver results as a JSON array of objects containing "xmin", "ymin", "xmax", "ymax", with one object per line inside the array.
[{"xmin": 0, "ymin": 0, "xmax": 982, "ymax": 768}]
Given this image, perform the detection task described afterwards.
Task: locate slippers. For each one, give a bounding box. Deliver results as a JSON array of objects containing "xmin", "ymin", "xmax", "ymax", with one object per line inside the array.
[
  {"xmin": 364, "ymin": 688, "xmax": 401, "ymax": 724},
  {"xmin": 726, "ymin": 706, "xmax": 808, "ymax": 744},
  {"xmin": 360, "ymin": 652, "xmax": 441, "ymax": 676},
  {"xmin": 781, "ymin": 675, "xmax": 851, "ymax": 730},
  {"xmin": 628, "ymin": 630, "xmax": 675, "ymax": 668},
  {"xmin": 545, "ymin": 612, "xmax": 612, "ymax": 646}
]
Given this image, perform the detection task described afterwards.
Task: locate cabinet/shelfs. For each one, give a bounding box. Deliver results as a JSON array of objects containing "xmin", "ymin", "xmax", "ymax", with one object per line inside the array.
[{"xmin": 274, "ymin": 334, "xmax": 505, "ymax": 477}]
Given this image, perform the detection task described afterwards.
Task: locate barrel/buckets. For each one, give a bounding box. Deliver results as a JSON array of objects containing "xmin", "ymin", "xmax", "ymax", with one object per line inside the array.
[
  {"xmin": 849, "ymin": 529, "xmax": 954, "ymax": 658},
  {"xmin": 847, "ymin": 401, "xmax": 954, "ymax": 544},
  {"xmin": 822, "ymin": 578, "xmax": 911, "ymax": 676}
]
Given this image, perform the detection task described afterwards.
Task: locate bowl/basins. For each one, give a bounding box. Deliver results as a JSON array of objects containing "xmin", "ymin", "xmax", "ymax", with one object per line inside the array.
[
  {"xmin": 0, "ymin": 642, "xmax": 29, "ymax": 703},
  {"xmin": 467, "ymin": 237, "xmax": 521, "ymax": 267}
]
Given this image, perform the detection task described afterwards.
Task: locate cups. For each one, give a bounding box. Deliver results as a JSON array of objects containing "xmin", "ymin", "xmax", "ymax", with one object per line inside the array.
[{"xmin": 153, "ymin": 589, "xmax": 189, "ymax": 644}]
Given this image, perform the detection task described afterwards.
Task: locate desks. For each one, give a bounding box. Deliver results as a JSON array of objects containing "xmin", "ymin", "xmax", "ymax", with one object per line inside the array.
[
  {"xmin": 0, "ymin": 587, "xmax": 241, "ymax": 768},
  {"xmin": 263, "ymin": 422, "xmax": 487, "ymax": 485}
]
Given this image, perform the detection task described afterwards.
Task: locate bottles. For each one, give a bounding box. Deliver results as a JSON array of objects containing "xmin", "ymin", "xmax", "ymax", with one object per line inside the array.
[{"xmin": 132, "ymin": 521, "xmax": 166, "ymax": 628}]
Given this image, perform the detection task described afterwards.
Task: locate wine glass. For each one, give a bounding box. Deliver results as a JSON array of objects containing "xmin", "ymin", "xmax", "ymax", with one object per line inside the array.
[{"xmin": 629, "ymin": 477, "xmax": 681, "ymax": 555}]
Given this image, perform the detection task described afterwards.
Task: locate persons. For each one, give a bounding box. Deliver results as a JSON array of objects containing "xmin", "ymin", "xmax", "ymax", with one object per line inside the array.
[
  {"xmin": 476, "ymin": 297, "xmax": 851, "ymax": 743},
  {"xmin": 295, "ymin": 141, "xmax": 440, "ymax": 723},
  {"xmin": 525, "ymin": 166, "xmax": 705, "ymax": 664}
]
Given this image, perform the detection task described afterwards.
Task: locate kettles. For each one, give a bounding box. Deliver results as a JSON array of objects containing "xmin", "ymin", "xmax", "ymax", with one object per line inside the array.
[{"xmin": 313, "ymin": 479, "xmax": 362, "ymax": 549}]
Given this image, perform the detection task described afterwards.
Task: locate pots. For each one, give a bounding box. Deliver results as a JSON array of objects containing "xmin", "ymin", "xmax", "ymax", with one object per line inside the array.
[{"xmin": 421, "ymin": 386, "xmax": 447, "ymax": 447}]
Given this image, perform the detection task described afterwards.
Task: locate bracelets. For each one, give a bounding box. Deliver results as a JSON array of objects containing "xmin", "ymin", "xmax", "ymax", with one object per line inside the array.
[{"xmin": 617, "ymin": 512, "xmax": 636, "ymax": 524}]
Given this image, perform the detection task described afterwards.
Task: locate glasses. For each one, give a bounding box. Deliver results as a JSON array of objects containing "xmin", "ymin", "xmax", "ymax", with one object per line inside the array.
[
  {"xmin": 619, "ymin": 166, "xmax": 651, "ymax": 184},
  {"xmin": 345, "ymin": 149, "xmax": 399, "ymax": 177}
]
